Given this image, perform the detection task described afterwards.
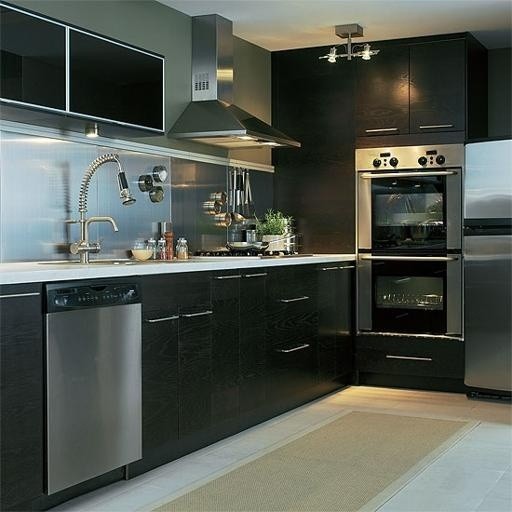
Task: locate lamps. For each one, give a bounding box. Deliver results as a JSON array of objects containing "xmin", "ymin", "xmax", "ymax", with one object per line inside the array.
[{"xmin": 315, "ymin": 21, "xmax": 381, "ymax": 65}]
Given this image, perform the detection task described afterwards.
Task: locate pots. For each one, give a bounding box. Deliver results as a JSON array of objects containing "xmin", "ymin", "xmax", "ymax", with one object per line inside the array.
[{"xmin": 225, "ymin": 234, "xmax": 297, "ymax": 250}]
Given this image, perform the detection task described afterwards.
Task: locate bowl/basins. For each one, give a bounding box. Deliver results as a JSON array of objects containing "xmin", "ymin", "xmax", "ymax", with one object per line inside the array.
[{"xmin": 130, "ymin": 249, "xmax": 153, "ymax": 261}]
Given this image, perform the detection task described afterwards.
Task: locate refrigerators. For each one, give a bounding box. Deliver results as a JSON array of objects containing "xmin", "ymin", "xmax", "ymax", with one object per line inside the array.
[{"xmin": 461, "ymin": 138, "xmax": 512, "ymax": 400}]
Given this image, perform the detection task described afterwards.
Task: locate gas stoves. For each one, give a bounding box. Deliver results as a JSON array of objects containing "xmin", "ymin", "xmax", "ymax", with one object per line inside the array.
[{"xmin": 193, "ymin": 250, "xmax": 313, "ymax": 260}]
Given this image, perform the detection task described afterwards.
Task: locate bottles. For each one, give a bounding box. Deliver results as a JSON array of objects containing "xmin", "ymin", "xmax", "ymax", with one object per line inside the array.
[
  {"xmin": 158, "ymin": 237, "xmax": 167, "ymax": 260},
  {"xmin": 147, "ymin": 238, "xmax": 157, "ymax": 259},
  {"xmin": 176, "ymin": 238, "xmax": 189, "ymax": 259}
]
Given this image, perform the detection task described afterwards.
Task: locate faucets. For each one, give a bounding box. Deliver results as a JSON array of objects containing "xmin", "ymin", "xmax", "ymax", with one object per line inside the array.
[{"xmin": 69, "ymin": 152, "xmax": 137, "ymax": 264}]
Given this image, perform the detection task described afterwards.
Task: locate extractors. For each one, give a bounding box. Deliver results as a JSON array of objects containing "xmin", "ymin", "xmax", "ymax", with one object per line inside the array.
[{"xmin": 166, "ymin": 14, "xmax": 303, "ymax": 151}]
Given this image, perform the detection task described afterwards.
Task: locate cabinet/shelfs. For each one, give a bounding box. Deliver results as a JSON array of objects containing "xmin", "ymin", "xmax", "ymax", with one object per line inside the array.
[
  {"xmin": 0, "ymin": 284, "xmax": 47, "ymax": 512},
  {"xmin": 356, "ymin": 332, "xmax": 464, "ymax": 396},
  {"xmin": 140, "ymin": 271, "xmax": 214, "ymax": 472},
  {"xmin": 318, "ymin": 256, "xmax": 354, "ymax": 402},
  {"xmin": 355, "ymin": 30, "xmax": 492, "ymax": 142},
  {"xmin": 213, "ymin": 266, "xmax": 266, "ymax": 441},
  {"xmin": 265, "ymin": 261, "xmax": 320, "ymax": 423}
]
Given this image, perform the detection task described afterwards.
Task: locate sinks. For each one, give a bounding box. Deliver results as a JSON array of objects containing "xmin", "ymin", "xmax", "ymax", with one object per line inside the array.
[{"xmin": 38, "ymin": 260, "xmax": 191, "ymax": 266}]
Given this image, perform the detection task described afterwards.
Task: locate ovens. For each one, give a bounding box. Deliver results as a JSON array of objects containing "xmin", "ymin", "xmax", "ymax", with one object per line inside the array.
[{"xmin": 354, "ymin": 144, "xmax": 464, "ymax": 339}]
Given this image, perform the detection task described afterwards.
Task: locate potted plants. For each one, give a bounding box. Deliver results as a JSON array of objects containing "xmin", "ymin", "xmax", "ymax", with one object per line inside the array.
[{"xmin": 254, "ymin": 206, "xmax": 296, "ymax": 258}]
